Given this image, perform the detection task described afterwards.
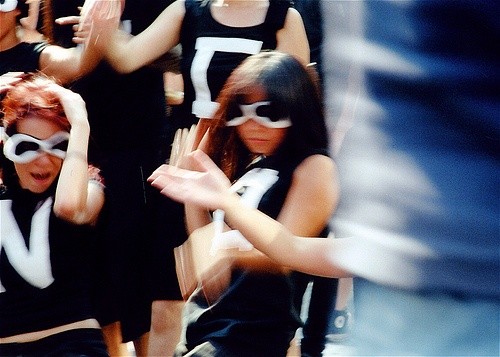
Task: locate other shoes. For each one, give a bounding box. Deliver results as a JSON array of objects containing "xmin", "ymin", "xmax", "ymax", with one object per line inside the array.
[{"xmin": 324, "ymin": 309, "xmax": 352, "ymax": 343}]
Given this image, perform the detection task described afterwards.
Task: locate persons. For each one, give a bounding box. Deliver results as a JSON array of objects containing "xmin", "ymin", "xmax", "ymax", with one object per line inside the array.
[
  {"xmin": 169, "ymin": 51, "xmax": 340, "ymax": 357},
  {"xmin": 0, "ymin": 71, "xmax": 107, "ymax": 357},
  {"xmin": 0, "ymin": 0, "xmax": 500, "ymax": 357}
]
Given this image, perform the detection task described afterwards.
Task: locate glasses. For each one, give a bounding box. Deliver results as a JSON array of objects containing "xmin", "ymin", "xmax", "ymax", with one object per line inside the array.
[
  {"xmin": 0, "ymin": 0, "xmax": 18, "ymax": 12},
  {"xmin": 2, "ymin": 130, "xmax": 70, "ymax": 164},
  {"xmin": 224, "ymin": 100, "xmax": 293, "ymax": 129}
]
